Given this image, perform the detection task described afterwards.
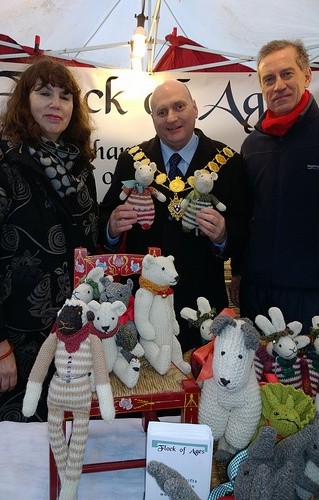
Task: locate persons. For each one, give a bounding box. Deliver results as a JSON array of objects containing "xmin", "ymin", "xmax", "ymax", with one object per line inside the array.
[
  {"xmin": 228, "ymin": 42, "xmax": 319, "ymax": 338},
  {"xmin": 96, "ymin": 81, "xmax": 251, "ymax": 354},
  {"xmin": 0, "ymin": 60, "xmax": 96, "ymax": 422}
]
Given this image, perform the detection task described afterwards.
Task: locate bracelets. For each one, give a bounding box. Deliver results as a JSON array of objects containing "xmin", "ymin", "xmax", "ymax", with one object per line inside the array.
[{"xmin": 0, "ymin": 344, "xmax": 13, "ymax": 360}]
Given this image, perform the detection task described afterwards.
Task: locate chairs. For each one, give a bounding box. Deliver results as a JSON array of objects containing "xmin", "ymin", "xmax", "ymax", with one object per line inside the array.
[{"xmin": 45, "ymin": 247, "xmax": 202, "ymax": 500}]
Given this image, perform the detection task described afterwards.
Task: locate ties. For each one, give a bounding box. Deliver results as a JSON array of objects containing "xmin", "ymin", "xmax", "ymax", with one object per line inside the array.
[{"xmin": 168, "ymin": 152, "xmax": 186, "ymax": 184}]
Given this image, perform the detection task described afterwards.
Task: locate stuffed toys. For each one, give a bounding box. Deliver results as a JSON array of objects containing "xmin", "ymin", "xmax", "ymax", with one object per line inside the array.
[
  {"xmin": 119, "ymin": 161, "xmax": 166, "ymax": 230},
  {"xmin": 21, "ymin": 299, "xmax": 115, "ymax": 500},
  {"xmin": 181, "ymin": 170, "xmax": 227, "ymax": 236},
  {"xmin": 147, "ymin": 297, "xmax": 319, "ymax": 500},
  {"xmin": 72, "ymin": 255, "xmax": 191, "ymax": 392}
]
